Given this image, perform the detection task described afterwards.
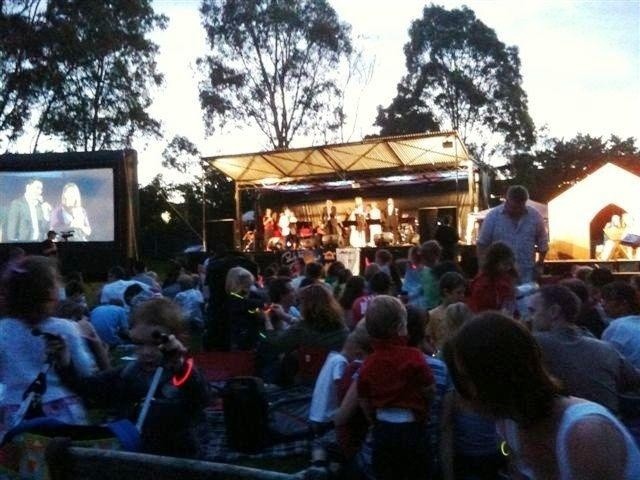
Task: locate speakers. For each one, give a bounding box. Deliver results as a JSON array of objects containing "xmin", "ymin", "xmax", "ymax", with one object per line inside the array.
[
  {"xmin": 218, "ymin": 218, "xmax": 234, "ymax": 251},
  {"xmin": 439, "ymin": 206, "xmax": 460, "ymax": 240},
  {"xmin": 204, "ymin": 219, "xmax": 218, "ymax": 250},
  {"xmin": 418, "ymin": 206, "xmax": 438, "ymax": 244}
]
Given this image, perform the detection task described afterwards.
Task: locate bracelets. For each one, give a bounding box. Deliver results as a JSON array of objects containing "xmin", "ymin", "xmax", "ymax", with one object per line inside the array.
[
  {"xmin": 501, "ymin": 441, "xmax": 510, "ymax": 457},
  {"xmin": 172, "ymin": 358, "xmax": 194, "ymax": 386}
]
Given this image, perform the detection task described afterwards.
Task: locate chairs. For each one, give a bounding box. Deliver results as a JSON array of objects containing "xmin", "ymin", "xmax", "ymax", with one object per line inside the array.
[{"xmin": 44, "ymin": 436, "xmax": 328, "ymax": 480}]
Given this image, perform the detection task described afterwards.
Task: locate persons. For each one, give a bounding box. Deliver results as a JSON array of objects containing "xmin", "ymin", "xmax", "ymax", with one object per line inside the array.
[
  {"xmin": 433, "ymin": 215, "xmax": 461, "ymax": 264},
  {"xmin": 247, "ymin": 195, "xmax": 403, "ymax": 248},
  {"xmin": 51, "ymin": 182, "xmax": 91, "ymax": 241},
  {"xmin": 0, "ymin": 230, "xmax": 640, "ymax": 480},
  {"xmin": 477, "ymin": 185, "xmax": 551, "ymax": 288},
  {"xmin": 6, "ymin": 180, "xmax": 53, "ymax": 243}
]
